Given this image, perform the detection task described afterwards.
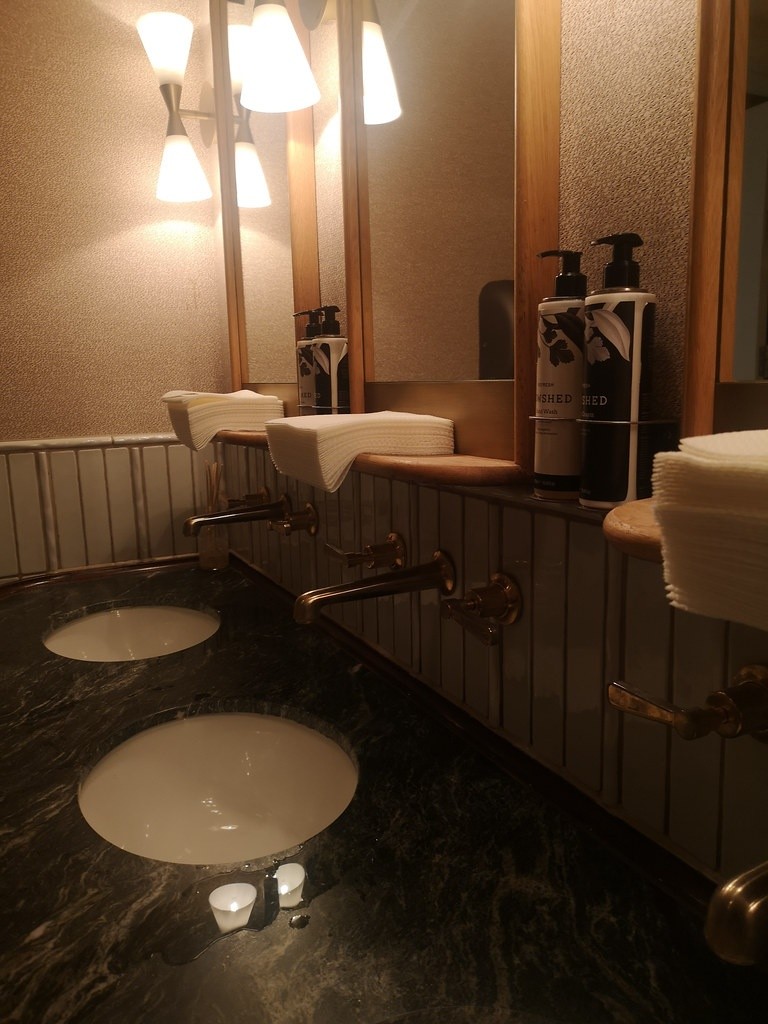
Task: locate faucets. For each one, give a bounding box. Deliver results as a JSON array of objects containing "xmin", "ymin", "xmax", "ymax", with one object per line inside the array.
[
  {"xmin": 292, "ymin": 546, "xmax": 460, "ymax": 627},
  {"xmin": 702, "ymin": 854, "xmax": 768, "ymax": 967},
  {"xmin": 180, "ymin": 494, "xmax": 293, "ymax": 540}
]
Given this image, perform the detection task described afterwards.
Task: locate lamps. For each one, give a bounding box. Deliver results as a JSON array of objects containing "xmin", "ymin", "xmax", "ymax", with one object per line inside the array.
[
  {"xmin": 224, "ymin": 1, "xmax": 316, "ymax": 213},
  {"xmin": 139, "ymin": 11, "xmax": 208, "ymax": 187},
  {"xmin": 360, "ymin": 1, "xmax": 403, "ymax": 126}
]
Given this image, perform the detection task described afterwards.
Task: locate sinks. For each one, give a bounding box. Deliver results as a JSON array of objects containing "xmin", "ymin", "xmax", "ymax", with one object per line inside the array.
[
  {"xmin": 40, "ymin": 597, "xmax": 221, "ymax": 665},
  {"xmin": 74, "ymin": 704, "xmax": 362, "ymax": 867}
]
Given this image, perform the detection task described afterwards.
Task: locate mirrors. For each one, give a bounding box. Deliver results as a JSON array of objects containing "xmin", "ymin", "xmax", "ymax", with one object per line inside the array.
[{"xmin": 211, "ymin": 0, "xmax": 768, "ymax": 500}]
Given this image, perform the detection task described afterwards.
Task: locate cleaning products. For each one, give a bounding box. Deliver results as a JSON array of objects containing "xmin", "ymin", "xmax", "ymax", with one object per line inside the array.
[
  {"xmin": 527, "ymin": 232, "xmax": 663, "ymax": 511},
  {"xmin": 290, "ymin": 305, "xmax": 351, "ymax": 415}
]
